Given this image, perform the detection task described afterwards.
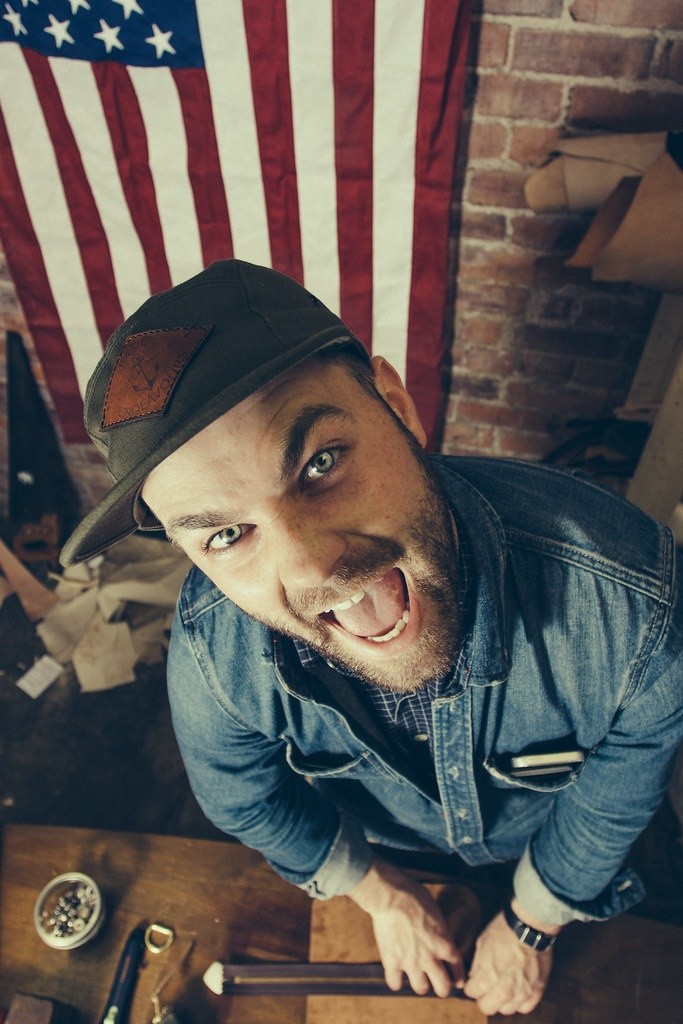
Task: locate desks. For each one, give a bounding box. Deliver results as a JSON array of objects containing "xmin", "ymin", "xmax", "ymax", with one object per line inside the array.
[{"xmin": 0, "ymin": 820, "xmax": 683, "ymax": 1023}]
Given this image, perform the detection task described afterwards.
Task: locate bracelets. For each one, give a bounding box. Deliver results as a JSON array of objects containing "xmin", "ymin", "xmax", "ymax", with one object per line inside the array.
[{"xmin": 504, "ymin": 906, "xmax": 556, "ymax": 953}]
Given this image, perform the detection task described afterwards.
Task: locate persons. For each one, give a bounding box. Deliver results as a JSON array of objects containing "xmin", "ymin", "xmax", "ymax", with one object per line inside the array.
[{"xmin": 60, "ymin": 259, "xmax": 683, "ymax": 1015}]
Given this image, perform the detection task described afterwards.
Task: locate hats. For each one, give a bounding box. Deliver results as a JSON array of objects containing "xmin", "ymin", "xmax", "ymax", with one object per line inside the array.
[{"xmin": 59, "ymin": 259, "xmax": 378, "ymax": 571}]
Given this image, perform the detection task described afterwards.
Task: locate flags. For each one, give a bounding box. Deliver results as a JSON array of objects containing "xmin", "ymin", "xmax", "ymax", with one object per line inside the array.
[{"xmin": 0, "ymin": 0, "xmax": 469, "ymax": 455}]
